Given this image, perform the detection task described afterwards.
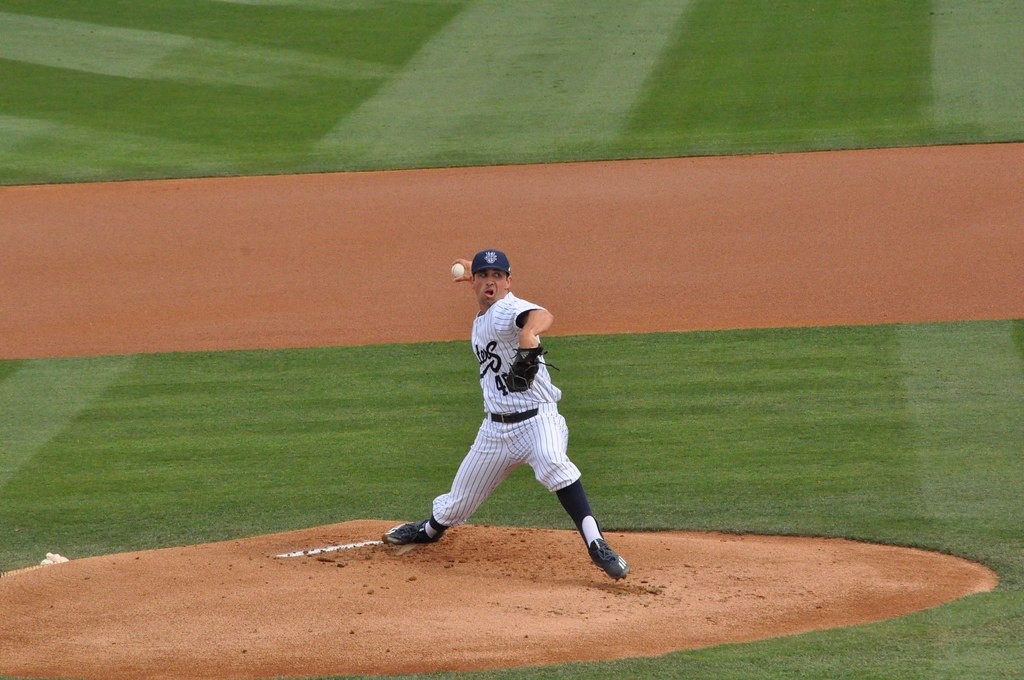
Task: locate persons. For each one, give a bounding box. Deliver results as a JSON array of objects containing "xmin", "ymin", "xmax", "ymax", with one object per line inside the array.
[{"xmin": 381, "ymin": 249, "xmax": 632, "ymax": 584}]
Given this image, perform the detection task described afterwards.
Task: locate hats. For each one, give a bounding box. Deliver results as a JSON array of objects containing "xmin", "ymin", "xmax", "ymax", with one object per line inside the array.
[{"xmin": 471, "ymin": 250, "xmax": 511, "ymax": 276}]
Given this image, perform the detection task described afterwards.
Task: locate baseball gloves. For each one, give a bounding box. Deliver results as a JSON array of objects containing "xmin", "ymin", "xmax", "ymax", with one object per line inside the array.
[{"xmin": 508, "ymin": 344, "xmax": 544, "ymax": 392}]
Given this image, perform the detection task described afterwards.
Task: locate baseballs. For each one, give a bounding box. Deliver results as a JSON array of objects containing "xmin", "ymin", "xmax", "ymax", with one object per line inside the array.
[{"xmin": 452, "ymin": 263, "xmax": 465, "ymax": 277}]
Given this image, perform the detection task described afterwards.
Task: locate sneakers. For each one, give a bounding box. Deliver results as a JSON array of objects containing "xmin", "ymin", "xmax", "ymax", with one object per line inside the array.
[
  {"xmin": 588, "ymin": 538, "xmax": 630, "ymax": 582},
  {"xmin": 381, "ymin": 519, "xmax": 444, "ymax": 544}
]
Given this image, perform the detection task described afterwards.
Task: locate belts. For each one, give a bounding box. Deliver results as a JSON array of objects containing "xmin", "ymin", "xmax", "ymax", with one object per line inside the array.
[{"xmin": 486, "ymin": 408, "xmax": 538, "ymax": 424}]
